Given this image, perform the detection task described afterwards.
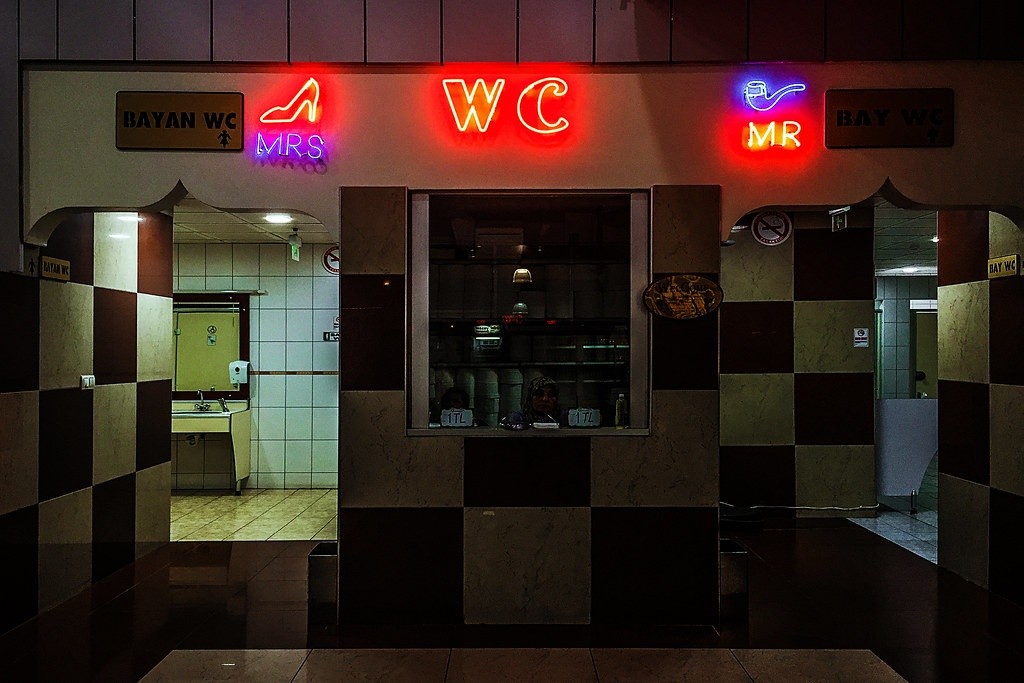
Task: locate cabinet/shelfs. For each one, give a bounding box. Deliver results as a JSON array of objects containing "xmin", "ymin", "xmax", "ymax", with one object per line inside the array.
[{"xmin": 405, "ymin": 187, "xmax": 655, "ymax": 436}]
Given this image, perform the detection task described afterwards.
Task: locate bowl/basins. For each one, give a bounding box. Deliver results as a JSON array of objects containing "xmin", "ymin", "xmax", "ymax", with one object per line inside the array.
[{"xmin": 429, "ymin": 368, "xmax": 523, "ymax": 427}]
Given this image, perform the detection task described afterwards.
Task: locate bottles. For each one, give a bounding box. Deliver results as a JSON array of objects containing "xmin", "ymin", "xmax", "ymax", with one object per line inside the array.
[{"xmin": 615, "ymin": 394, "xmax": 628, "ymax": 429}]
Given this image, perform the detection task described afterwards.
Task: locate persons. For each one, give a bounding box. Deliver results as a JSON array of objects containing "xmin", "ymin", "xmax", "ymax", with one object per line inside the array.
[
  {"xmin": 499, "ymin": 376, "xmax": 582, "ymax": 428},
  {"xmin": 429, "ymin": 388, "xmax": 487, "ymax": 423}
]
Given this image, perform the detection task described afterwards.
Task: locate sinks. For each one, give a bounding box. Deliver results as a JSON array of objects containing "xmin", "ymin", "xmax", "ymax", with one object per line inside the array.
[{"xmin": 172, "ymin": 410, "xmax": 224, "ymax": 415}]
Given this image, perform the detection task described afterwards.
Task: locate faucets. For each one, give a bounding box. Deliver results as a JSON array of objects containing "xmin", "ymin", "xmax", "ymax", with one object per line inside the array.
[{"xmin": 194, "ymin": 388, "xmax": 211, "ymax": 411}]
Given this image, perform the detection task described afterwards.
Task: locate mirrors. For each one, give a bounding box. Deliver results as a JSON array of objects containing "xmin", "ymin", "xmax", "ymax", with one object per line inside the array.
[
  {"xmin": 875, "ymin": 276, "xmax": 939, "ymax": 400},
  {"xmin": 173, "ymin": 313, "xmax": 240, "ymax": 392}
]
